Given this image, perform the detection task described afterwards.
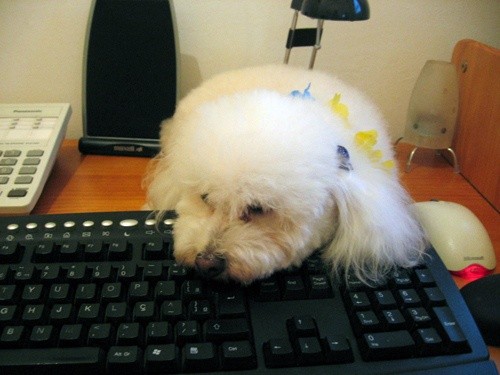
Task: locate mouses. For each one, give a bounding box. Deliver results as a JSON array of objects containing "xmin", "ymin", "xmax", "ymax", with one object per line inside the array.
[{"xmin": 409, "ymin": 199, "xmax": 496, "ymax": 272}]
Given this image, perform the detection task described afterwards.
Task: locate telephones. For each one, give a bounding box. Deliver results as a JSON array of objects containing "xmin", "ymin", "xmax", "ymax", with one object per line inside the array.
[{"xmin": 0, "ymin": 104, "xmax": 73, "ymax": 217}]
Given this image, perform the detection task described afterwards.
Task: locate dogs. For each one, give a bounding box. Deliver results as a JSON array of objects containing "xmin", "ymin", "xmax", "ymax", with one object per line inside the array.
[{"xmin": 140, "ymin": 64, "xmax": 428, "ymax": 286}]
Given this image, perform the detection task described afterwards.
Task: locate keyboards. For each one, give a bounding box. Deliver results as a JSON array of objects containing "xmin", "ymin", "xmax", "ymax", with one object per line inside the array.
[{"xmin": 0, "ymin": 210, "xmax": 500, "ymax": 374}]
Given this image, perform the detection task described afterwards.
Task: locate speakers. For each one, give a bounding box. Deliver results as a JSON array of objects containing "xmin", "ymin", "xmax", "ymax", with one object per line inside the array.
[{"xmin": 74, "ymin": 0, "xmax": 178, "ymax": 159}]
